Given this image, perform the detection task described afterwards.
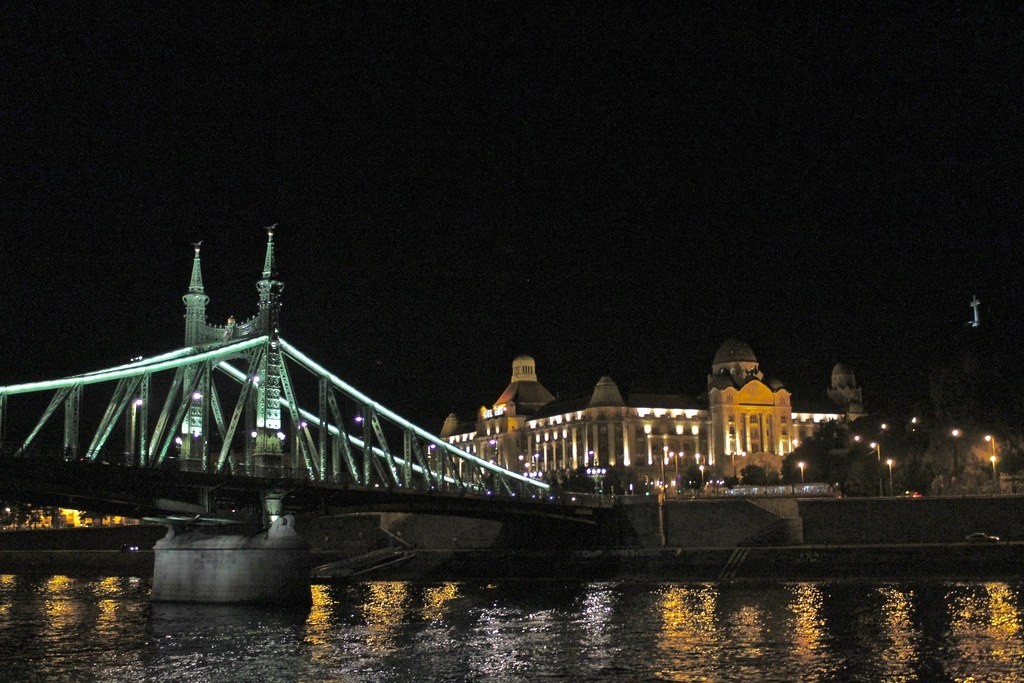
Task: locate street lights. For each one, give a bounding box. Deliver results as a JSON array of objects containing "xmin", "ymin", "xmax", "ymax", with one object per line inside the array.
[
  {"xmin": 886, "ymin": 460, "xmax": 894, "ymax": 496},
  {"xmin": 985, "ymin": 435, "xmax": 998, "ymax": 491},
  {"xmin": 700, "ymin": 465, "xmax": 705, "ymax": 484},
  {"xmin": 798, "ymin": 462, "xmax": 805, "ymax": 491},
  {"xmin": 870, "ymin": 442, "xmax": 884, "ymax": 495}
]
